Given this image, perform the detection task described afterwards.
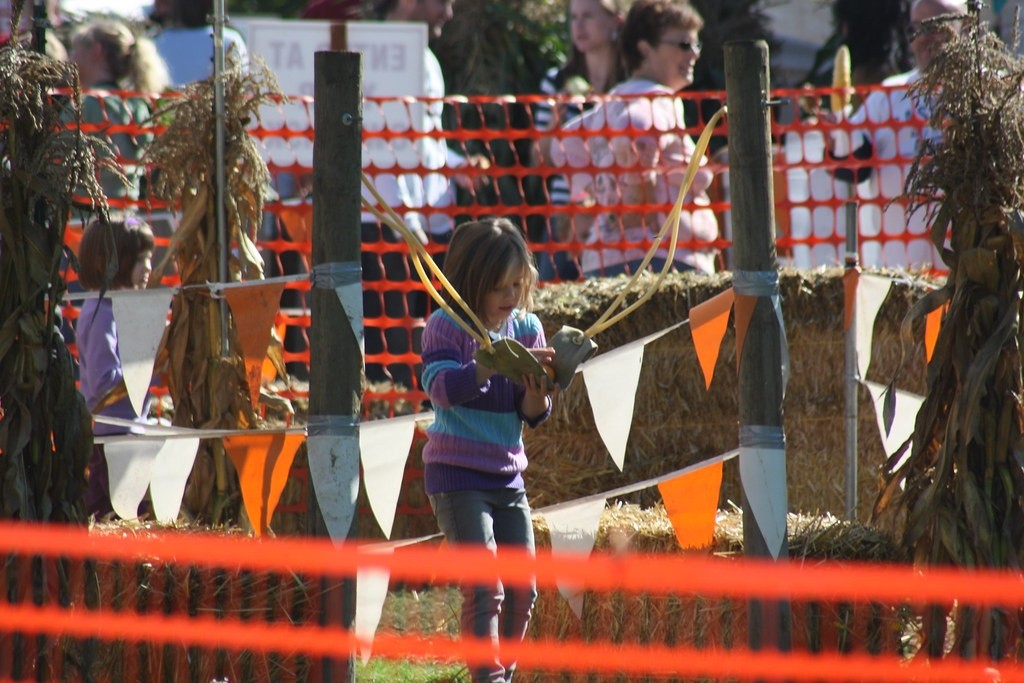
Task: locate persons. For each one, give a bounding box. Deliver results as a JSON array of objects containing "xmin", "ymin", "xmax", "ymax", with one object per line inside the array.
[
  {"xmin": 0, "ymin": 0, "xmax": 166, "ymax": 381},
  {"xmin": 817, "ymin": 0, "xmax": 1024, "ymax": 491},
  {"xmin": 533, "ymin": 1, "xmax": 721, "ymax": 284},
  {"xmin": 140, "ymin": 0, "xmax": 249, "ymax": 286},
  {"xmin": 75, "ymin": 214, "xmax": 174, "ymax": 435},
  {"xmin": 253, "ymin": 172, "xmax": 314, "ymax": 381},
  {"xmin": 423, "ymin": 217, "xmax": 555, "ymax": 682},
  {"xmin": 361, "ymin": 0, "xmax": 492, "ymax": 389}
]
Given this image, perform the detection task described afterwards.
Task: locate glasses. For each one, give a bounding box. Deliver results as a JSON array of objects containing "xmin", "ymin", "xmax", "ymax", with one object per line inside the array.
[{"xmin": 654, "ymin": 38, "xmax": 704, "ymax": 54}]
